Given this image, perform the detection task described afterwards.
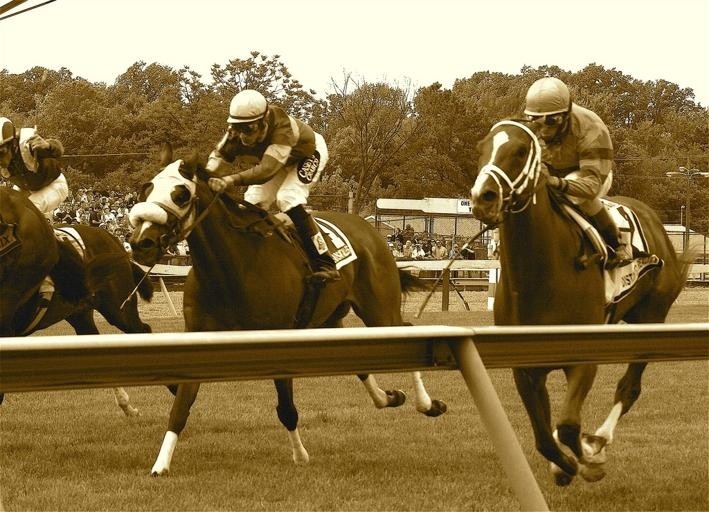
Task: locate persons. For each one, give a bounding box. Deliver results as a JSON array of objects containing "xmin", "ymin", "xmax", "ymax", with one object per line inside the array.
[
  {"xmin": 50, "ymin": 185, "xmax": 189, "ymax": 257},
  {"xmin": 207, "ymin": 90, "xmax": 343, "ymax": 284},
  {"xmin": 1, "ymin": 118, "xmax": 89, "ymax": 265},
  {"xmin": 386, "ymin": 223, "xmax": 475, "ymax": 278},
  {"xmin": 522, "ymin": 74, "xmax": 638, "ymax": 270}
]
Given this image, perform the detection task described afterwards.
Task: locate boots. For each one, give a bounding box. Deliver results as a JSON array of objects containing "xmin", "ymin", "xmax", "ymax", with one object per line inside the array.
[
  {"xmin": 600, "ymin": 221, "xmax": 623, "ymax": 269},
  {"xmin": 296, "ymin": 215, "xmax": 343, "ymax": 283}
]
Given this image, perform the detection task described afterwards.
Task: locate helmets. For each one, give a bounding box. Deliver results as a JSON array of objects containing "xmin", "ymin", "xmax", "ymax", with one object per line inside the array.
[
  {"xmin": 524, "ymin": 76, "xmax": 572, "ymax": 116},
  {"xmin": 227, "ymin": 90, "xmax": 267, "ymax": 123},
  {"xmin": 0, "ymin": 117, "xmax": 16, "ymax": 145}
]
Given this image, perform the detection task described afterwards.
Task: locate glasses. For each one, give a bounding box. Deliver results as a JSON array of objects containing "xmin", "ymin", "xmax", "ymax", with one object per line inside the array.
[
  {"xmin": 0, "ymin": 143, "xmax": 11, "ymax": 154},
  {"xmin": 532, "ymin": 117, "xmax": 558, "ymax": 128},
  {"xmin": 232, "ymin": 124, "xmax": 261, "ymax": 134}
]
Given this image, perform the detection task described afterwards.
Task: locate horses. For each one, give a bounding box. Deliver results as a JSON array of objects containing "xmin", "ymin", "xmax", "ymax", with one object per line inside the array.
[
  {"xmin": 128, "ymin": 151, "xmax": 448, "ymax": 478},
  {"xmin": 0, "ymin": 185, "xmax": 181, "ymax": 418},
  {"xmin": 469, "ymin": 115, "xmax": 709, "ymax": 487}
]
